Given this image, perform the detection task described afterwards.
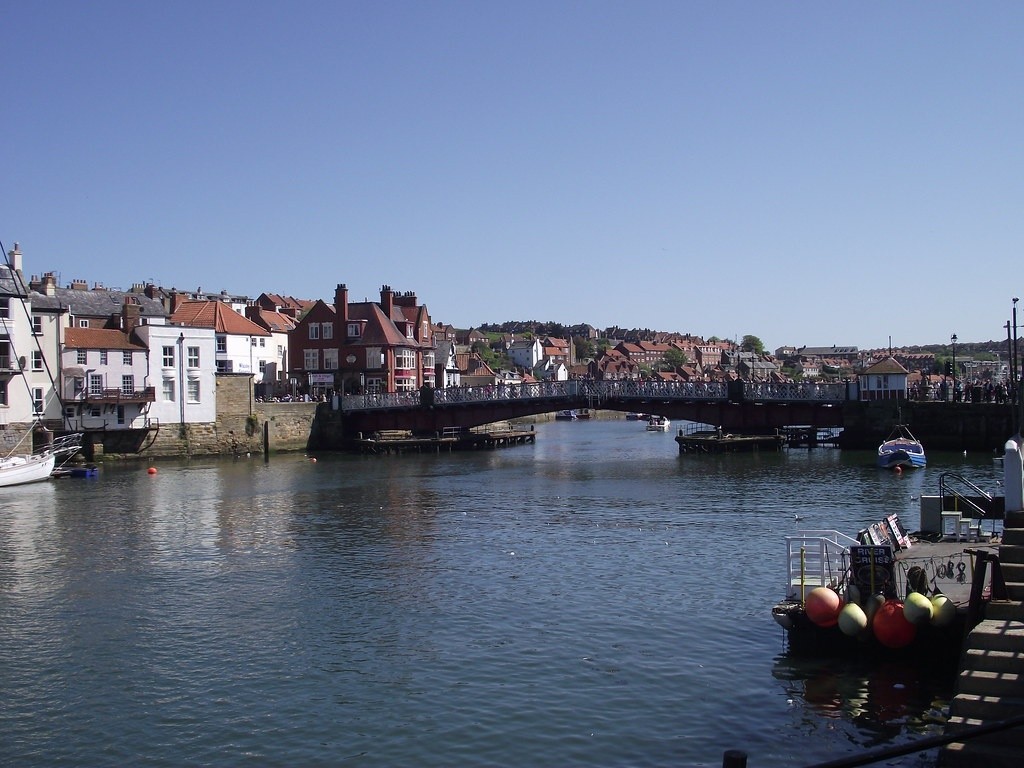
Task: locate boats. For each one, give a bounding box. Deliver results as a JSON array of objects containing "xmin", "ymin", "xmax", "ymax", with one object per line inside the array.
[
  {"xmin": 675, "ymin": 422, "xmax": 786, "ymax": 452},
  {"xmin": 626, "ymin": 412, "xmax": 660, "ymax": 420},
  {"xmin": 555, "ymin": 409, "xmax": 590, "ymax": 420},
  {"xmin": 878, "ymin": 437, "xmax": 926, "ymax": 468},
  {"xmin": 645, "ymin": 417, "xmax": 671, "ymax": 432},
  {"xmin": 0, "ymin": 450, "xmax": 55, "ymax": 486},
  {"xmin": 71, "ymin": 464, "xmax": 98, "ymax": 479}
]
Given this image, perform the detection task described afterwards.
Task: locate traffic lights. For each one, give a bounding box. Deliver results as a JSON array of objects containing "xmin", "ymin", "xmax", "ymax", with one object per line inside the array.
[{"xmin": 946, "ymin": 361, "xmax": 952, "ymax": 376}]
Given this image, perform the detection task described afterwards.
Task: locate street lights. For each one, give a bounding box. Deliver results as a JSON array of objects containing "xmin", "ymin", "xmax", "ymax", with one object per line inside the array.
[
  {"xmin": 752, "ymin": 348, "xmax": 755, "ymax": 380},
  {"xmin": 737, "ymin": 346, "xmax": 740, "ymax": 379},
  {"xmin": 950, "ymin": 333, "xmax": 958, "ymax": 388}
]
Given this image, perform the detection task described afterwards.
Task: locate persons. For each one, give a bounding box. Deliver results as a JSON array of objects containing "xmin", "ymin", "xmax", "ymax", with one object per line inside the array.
[
  {"xmin": 256, "ymin": 394, "xmax": 327, "ymax": 402},
  {"xmin": 910, "ymin": 380, "xmax": 1024, "ymax": 405},
  {"xmin": 584, "ymin": 377, "xmax": 726, "ymax": 396},
  {"xmin": 435, "ymin": 379, "xmax": 570, "ymax": 402},
  {"xmin": 329, "ymin": 389, "xmax": 420, "ymax": 406},
  {"xmin": 742, "ymin": 378, "xmax": 836, "ymax": 398}
]
